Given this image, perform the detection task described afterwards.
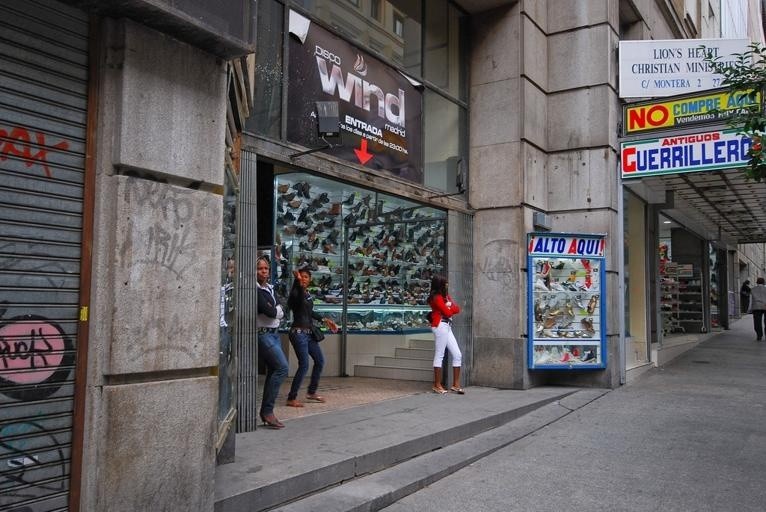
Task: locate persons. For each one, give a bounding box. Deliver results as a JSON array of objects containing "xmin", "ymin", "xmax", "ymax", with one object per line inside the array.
[
  {"xmin": 257, "ymin": 251, "xmax": 290, "ymax": 428},
  {"xmin": 427, "ymin": 272, "xmax": 465, "ymax": 394},
  {"xmin": 220, "ymin": 259, "xmax": 238, "ymax": 411},
  {"xmin": 740, "ymin": 280, "xmax": 751, "ymax": 313},
  {"xmin": 286, "ymin": 268, "xmax": 340, "ymax": 408},
  {"xmin": 749, "ymin": 277, "xmax": 766, "ymax": 340}
]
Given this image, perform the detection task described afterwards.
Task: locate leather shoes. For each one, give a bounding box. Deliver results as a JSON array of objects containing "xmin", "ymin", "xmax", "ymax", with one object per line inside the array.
[
  {"xmin": 305, "ymin": 392, "xmax": 324, "ymax": 401},
  {"xmin": 534, "ymin": 261, "xmax": 596, "ymax": 363},
  {"xmin": 286, "ymin": 400, "xmax": 303, "ymax": 408},
  {"xmin": 274, "ymin": 181, "xmax": 448, "ymax": 330}
]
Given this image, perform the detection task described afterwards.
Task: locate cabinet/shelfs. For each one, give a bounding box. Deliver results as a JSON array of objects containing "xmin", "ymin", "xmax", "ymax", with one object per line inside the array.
[
  {"xmin": 271, "ymin": 171, "xmax": 447, "ymax": 332},
  {"xmin": 657, "ymin": 263, "xmax": 706, "ymax": 338}
]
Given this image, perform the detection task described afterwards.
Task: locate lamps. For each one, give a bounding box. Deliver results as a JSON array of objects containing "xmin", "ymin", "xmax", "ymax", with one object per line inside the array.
[
  {"xmin": 288, "ymin": 98, "xmax": 345, "ymax": 161},
  {"xmin": 425, "ymin": 154, "xmax": 468, "ymax": 201}
]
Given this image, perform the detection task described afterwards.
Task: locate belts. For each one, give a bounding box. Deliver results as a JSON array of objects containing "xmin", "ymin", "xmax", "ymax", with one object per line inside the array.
[{"xmin": 258, "ymin": 327, "xmax": 278, "ymax": 333}]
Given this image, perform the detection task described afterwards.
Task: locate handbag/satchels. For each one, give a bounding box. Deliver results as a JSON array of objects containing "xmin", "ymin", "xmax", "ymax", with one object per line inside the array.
[{"xmin": 310, "ymin": 322, "xmax": 324, "ymax": 342}]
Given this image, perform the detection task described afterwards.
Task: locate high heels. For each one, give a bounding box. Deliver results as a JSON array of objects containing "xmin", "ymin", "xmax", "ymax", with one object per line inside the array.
[
  {"xmin": 450, "ymin": 386, "xmax": 463, "ymax": 395},
  {"xmin": 432, "ymin": 386, "xmax": 447, "ymax": 394},
  {"xmin": 260, "ymin": 414, "xmax": 285, "ymax": 428}
]
